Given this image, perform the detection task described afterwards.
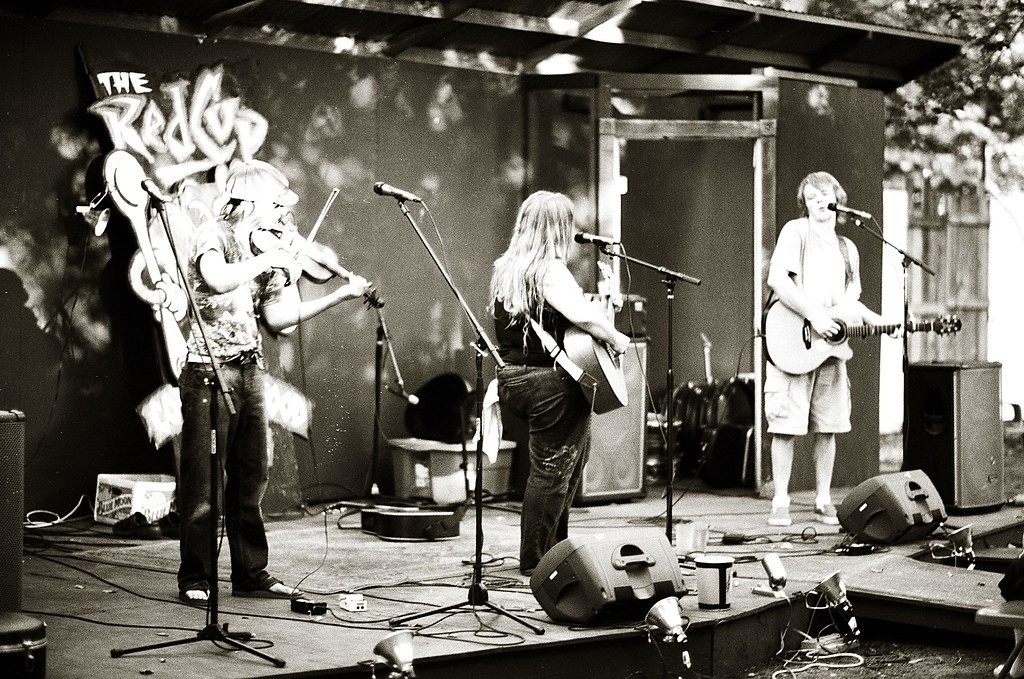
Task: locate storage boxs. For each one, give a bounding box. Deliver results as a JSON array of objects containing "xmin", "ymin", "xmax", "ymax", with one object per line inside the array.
[
  {"xmin": 94, "ymin": 474, "xmax": 179, "ymax": 527},
  {"xmin": 389, "ymin": 435, "xmax": 518, "ymax": 503}
]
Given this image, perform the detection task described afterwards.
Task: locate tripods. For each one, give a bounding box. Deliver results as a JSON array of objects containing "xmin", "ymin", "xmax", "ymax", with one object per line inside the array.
[
  {"xmin": 111, "ymin": 198, "xmax": 287, "ymax": 668},
  {"xmin": 389, "ymin": 200, "xmax": 546, "ymax": 636}
]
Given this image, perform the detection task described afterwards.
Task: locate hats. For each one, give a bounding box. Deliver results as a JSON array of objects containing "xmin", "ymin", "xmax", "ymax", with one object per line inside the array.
[{"xmin": 226, "ymin": 159, "xmax": 299, "ymax": 206}]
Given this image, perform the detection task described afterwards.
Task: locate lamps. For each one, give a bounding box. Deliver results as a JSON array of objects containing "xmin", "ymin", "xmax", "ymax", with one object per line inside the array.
[
  {"xmin": 931, "ymin": 524, "xmax": 976, "ymax": 572},
  {"xmin": 811, "ymin": 570, "xmax": 862, "ymax": 639},
  {"xmin": 644, "ymin": 596, "xmax": 692, "ymax": 669},
  {"xmin": 357, "ymin": 630, "xmax": 416, "ymax": 679}
]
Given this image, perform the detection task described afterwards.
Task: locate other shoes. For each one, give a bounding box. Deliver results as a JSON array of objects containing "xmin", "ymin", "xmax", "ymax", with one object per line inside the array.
[
  {"xmin": 813, "ymin": 502, "xmax": 839, "ymax": 524},
  {"xmin": 768, "ymin": 507, "xmax": 791, "ymax": 525}
]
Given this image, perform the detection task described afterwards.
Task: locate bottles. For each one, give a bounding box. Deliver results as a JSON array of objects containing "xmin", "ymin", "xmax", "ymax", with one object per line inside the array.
[{"xmin": 371, "ymin": 483, "xmax": 379, "ymax": 495}]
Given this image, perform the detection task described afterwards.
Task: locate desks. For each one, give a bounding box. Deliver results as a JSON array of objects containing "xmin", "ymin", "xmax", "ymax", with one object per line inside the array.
[{"xmin": 570, "ymin": 335, "xmax": 652, "ymax": 505}]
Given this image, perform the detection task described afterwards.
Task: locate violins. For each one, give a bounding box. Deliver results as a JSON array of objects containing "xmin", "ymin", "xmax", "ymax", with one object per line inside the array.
[{"xmin": 249, "ymin": 220, "xmax": 387, "ymax": 309}]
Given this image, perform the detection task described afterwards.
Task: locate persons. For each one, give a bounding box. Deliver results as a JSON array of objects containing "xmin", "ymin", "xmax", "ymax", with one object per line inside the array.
[
  {"xmin": 176, "ymin": 159, "xmax": 372, "ymax": 607},
  {"xmin": 486, "ymin": 190, "xmax": 631, "ymax": 578},
  {"xmin": 767, "ymin": 169, "xmax": 908, "ymax": 527}
]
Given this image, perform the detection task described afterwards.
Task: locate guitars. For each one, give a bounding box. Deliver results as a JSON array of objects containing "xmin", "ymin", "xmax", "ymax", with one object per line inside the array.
[
  {"xmin": 761, "ymin": 298, "xmax": 962, "ymax": 377},
  {"xmin": 562, "ymin": 261, "xmax": 628, "ymax": 415}
]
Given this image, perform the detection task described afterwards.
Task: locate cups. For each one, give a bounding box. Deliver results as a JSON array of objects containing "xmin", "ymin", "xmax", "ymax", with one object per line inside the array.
[{"xmin": 694, "ymin": 557, "xmax": 736, "ymax": 611}]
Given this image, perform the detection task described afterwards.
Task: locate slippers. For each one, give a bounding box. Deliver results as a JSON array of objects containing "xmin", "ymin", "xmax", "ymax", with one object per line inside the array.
[
  {"xmin": 179, "ymin": 581, "xmax": 211, "ymax": 606},
  {"xmin": 232, "ymin": 576, "xmax": 304, "ymax": 599}
]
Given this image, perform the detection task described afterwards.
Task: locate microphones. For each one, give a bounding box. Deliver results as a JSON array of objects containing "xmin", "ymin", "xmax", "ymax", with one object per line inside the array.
[
  {"xmin": 384, "ymin": 384, "xmax": 420, "ymax": 405},
  {"xmin": 828, "ymin": 203, "xmax": 873, "ymax": 220},
  {"xmin": 574, "ymin": 232, "xmax": 621, "ymax": 245},
  {"xmin": 141, "ymin": 177, "xmax": 166, "ymax": 203},
  {"xmin": 373, "ymin": 182, "xmax": 422, "ymax": 203}
]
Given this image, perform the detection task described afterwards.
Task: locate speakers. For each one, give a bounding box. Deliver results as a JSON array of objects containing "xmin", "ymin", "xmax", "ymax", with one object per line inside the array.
[
  {"xmin": 568, "ymin": 337, "xmax": 653, "ymax": 505},
  {"xmin": 907, "ymin": 361, "xmax": 1005, "ymax": 517},
  {"xmin": 0, "ymin": 408, "xmax": 25, "ymax": 616},
  {"xmin": 837, "ymin": 469, "xmax": 948, "ymax": 546},
  {"xmin": 529, "ymin": 527, "xmax": 689, "ymax": 628}
]
